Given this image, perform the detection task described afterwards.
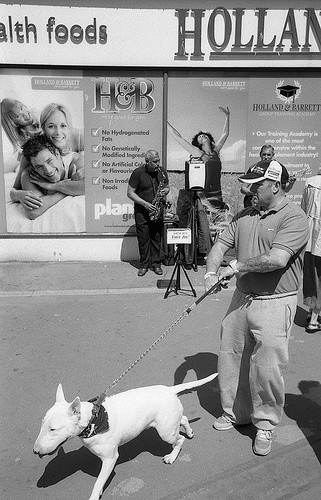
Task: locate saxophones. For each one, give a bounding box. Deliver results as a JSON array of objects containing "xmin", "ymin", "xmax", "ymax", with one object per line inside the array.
[{"xmin": 148, "ymin": 165, "xmax": 170, "ymax": 221}]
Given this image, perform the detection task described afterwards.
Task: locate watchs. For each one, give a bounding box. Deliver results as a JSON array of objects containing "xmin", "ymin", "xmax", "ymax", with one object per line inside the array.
[{"xmin": 229, "ymin": 264, "xmax": 239, "ymax": 274}]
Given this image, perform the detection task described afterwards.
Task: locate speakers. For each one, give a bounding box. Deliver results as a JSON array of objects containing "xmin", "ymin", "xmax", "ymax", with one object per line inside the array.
[{"xmin": 186, "ymin": 161, "xmax": 207, "ymax": 191}]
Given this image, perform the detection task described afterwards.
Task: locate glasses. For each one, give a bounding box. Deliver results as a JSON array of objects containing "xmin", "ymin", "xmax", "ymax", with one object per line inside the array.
[
  {"xmin": 152, "ymin": 158, "xmax": 160, "ymax": 163},
  {"xmin": 262, "ymin": 153, "xmax": 272, "ymax": 156}
]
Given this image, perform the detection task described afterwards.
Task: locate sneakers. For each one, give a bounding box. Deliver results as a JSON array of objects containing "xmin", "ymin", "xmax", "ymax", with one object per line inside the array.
[
  {"xmin": 253, "ymin": 428, "xmax": 273, "ymax": 456},
  {"xmin": 213, "ymin": 414, "xmax": 250, "ymax": 430}
]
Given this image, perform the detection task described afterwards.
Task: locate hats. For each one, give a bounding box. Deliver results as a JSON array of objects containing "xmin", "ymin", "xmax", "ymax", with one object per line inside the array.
[{"xmin": 238, "ymin": 159, "xmax": 289, "ymax": 185}]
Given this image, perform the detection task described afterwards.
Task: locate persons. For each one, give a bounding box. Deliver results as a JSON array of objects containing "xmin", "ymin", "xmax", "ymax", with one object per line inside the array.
[
  {"xmin": 300, "ymin": 170, "xmax": 321, "ymax": 333},
  {"xmin": 0, "ymin": 98, "xmax": 85, "ymax": 221},
  {"xmin": 127, "ymin": 150, "xmax": 170, "ymax": 277},
  {"xmin": 167, "ymin": 105, "xmax": 231, "ymax": 214},
  {"xmin": 241, "ymin": 145, "xmax": 297, "ymax": 196},
  {"xmin": 203, "ymin": 158, "xmax": 310, "ymax": 456}
]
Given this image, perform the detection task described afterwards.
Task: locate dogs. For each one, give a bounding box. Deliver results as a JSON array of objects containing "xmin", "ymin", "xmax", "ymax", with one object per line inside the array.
[{"xmin": 30, "ymin": 368, "xmax": 221, "ymax": 500}]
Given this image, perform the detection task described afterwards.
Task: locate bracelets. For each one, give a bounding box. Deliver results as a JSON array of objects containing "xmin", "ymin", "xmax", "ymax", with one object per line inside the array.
[{"xmin": 204, "ymin": 272, "xmax": 217, "ymax": 280}]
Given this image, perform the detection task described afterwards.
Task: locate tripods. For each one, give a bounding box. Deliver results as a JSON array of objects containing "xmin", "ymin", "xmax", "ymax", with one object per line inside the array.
[{"xmin": 164, "ymin": 247, "xmax": 197, "ymax": 299}]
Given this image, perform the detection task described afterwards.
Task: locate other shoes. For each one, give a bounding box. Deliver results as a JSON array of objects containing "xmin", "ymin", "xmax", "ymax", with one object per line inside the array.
[
  {"xmin": 153, "ymin": 267, "xmax": 163, "ymax": 275},
  {"xmin": 137, "ymin": 268, "xmax": 148, "ymax": 276},
  {"xmin": 306, "ymin": 323, "xmax": 321, "ymax": 332}
]
